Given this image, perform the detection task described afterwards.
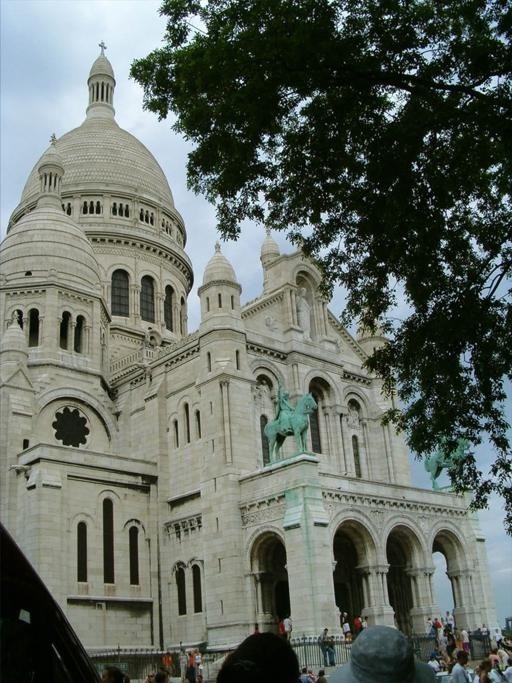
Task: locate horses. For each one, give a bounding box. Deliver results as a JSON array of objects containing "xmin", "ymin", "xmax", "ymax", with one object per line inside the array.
[{"xmin": 263, "ymin": 391, "xmax": 318, "ymax": 464}]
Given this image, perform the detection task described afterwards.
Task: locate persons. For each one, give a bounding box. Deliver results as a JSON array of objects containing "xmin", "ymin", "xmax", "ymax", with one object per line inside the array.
[
  {"xmin": 351, "ymin": 406, "xmax": 360, "ymax": 424},
  {"xmin": 102, "ymin": 665, "xmax": 131, "ymax": 683},
  {"xmin": 300, "ymin": 669, "xmax": 327, "ymax": 683},
  {"xmin": 278, "ymin": 615, "xmax": 292, "ymax": 640},
  {"xmin": 425, "ymin": 610, "xmax": 512, "ymax": 683},
  {"xmin": 347, "ymin": 402, "xmax": 353, "ymax": 423},
  {"xmin": 342, "ymin": 612, "xmax": 368, "ymax": 641},
  {"xmin": 319, "ymin": 628, "xmax": 336, "ymax": 667},
  {"xmin": 259, "ymin": 380, "xmax": 271, "ymax": 396},
  {"xmin": 280, "ymin": 392, "xmax": 294, "ymax": 433},
  {"xmin": 255, "ymin": 379, "xmax": 261, "ymax": 396},
  {"xmin": 146, "ymin": 648, "xmax": 205, "ymax": 683}
]
